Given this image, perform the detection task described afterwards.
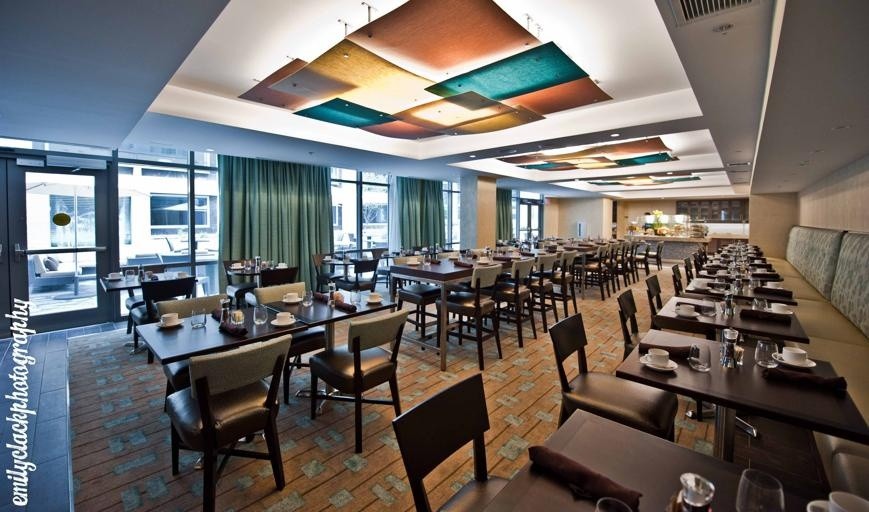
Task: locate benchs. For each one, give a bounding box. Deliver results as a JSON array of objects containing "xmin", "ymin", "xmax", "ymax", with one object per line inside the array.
[
  {"xmin": 792, "ymin": 228, "xmax": 868, "ymax": 500},
  {"xmin": 762, "ymin": 224, "xmax": 849, "ymax": 302}
]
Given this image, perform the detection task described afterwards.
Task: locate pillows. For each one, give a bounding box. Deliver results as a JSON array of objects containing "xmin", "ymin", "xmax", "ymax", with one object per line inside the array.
[{"xmin": 43, "ymin": 256, "xmax": 60, "ymax": 272}]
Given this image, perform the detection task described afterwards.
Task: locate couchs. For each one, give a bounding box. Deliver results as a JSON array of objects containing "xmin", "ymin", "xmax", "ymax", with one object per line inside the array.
[{"xmin": 30, "ymin": 253, "xmax": 82, "ymax": 288}]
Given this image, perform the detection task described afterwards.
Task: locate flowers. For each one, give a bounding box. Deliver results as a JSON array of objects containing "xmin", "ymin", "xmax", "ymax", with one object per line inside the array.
[{"xmin": 652, "ymin": 209, "xmax": 671, "ymax": 236}]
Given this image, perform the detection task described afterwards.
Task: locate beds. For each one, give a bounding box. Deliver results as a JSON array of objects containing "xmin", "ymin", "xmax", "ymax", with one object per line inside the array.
[{"xmin": 134, "ymin": 304, "xmax": 308, "ymax": 413}]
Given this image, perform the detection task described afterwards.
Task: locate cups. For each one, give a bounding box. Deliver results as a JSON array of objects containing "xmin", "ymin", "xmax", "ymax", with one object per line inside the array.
[
  {"xmin": 827, "ymin": 489, "xmax": 869, "ymax": 512},
  {"xmin": 780, "ymin": 346, "xmax": 808, "ymax": 365},
  {"xmin": 687, "ymin": 341, "xmax": 711, "ymax": 372},
  {"xmin": 302, "ymin": 290, "xmax": 312, "ymax": 306},
  {"xmin": 369, "ymin": 293, "xmax": 380, "ymax": 302},
  {"xmin": 643, "ymin": 348, "xmax": 669, "ymax": 366},
  {"xmin": 107, "ymin": 261, "xmax": 189, "ymax": 282},
  {"xmin": 323, "ymin": 247, "xmax": 367, "ymax": 264},
  {"xmin": 366, "ymin": 233, "xmax": 546, "ymax": 266},
  {"xmin": 276, "ymin": 311, "xmax": 294, "ymax": 323},
  {"xmin": 254, "ymin": 305, "xmax": 268, "ymax": 325},
  {"xmin": 283, "ymin": 293, "xmax": 298, "ymax": 301},
  {"xmin": 350, "ymin": 289, "xmax": 361, "ymax": 305},
  {"xmin": 734, "ymin": 467, "xmax": 786, "ymax": 512},
  {"xmin": 676, "ymin": 239, "xmax": 787, "ymax": 317},
  {"xmin": 594, "ymin": 496, "xmax": 632, "ymax": 512},
  {"xmin": 228, "ymin": 253, "xmax": 290, "ymax": 273},
  {"xmin": 753, "ymin": 340, "xmax": 779, "ymax": 368},
  {"xmin": 161, "ymin": 312, "xmax": 178, "ymax": 324},
  {"xmin": 191, "ymin": 308, "xmax": 207, "ymax": 328}
]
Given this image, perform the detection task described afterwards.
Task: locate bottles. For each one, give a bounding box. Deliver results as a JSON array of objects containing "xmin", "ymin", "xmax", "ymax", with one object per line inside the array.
[
  {"xmin": 720, "ymin": 327, "xmax": 738, "ymax": 369},
  {"xmin": 218, "ymin": 297, "xmax": 231, "ymax": 326},
  {"xmin": 328, "ymin": 281, "xmax": 336, "ymax": 307},
  {"xmin": 665, "ymin": 472, "xmax": 718, "ymax": 512}
]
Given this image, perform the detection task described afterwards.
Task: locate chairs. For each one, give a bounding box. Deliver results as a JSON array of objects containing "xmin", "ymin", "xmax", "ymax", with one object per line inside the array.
[
  {"xmin": 164, "ymin": 334, "xmax": 295, "ymax": 512},
  {"xmin": 306, "ymin": 307, "xmax": 410, "ymax": 453},
  {"xmin": 547, "ymin": 312, "xmax": 679, "ymax": 443},
  {"xmin": 100, "ymin": 244, "xmax": 308, "ymax": 365},
  {"xmin": 391, "ymin": 372, "xmax": 514, "ymax": 511},
  {"xmin": 309, "ymin": 238, "xmax": 811, "ymax": 369}
]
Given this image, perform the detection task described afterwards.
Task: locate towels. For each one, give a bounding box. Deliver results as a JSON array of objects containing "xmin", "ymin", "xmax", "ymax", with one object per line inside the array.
[
  {"xmin": 526, "ymin": 445, "xmax": 643, "ymax": 511},
  {"xmin": 761, "ymin": 367, "xmax": 847, "ymax": 398}
]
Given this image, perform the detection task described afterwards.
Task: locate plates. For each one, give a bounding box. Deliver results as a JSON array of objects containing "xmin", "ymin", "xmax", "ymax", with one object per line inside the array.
[
  {"xmin": 772, "ymin": 352, "xmax": 816, "ymax": 367},
  {"xmin": 764, "ymin": 308, "xmax": 794, "ymax": 314},
  {"xmin": 282, "ymin": 298, "xmax": 302, "ymax": 304},
  {"xmin": 639, "ymin": 354, "xmax": 678, "ymax": 373},
  {"xmin": 271, "ymin": 318, "xmax": 296, "ymax": 327},
  {"xmin": 366, "ymin": 298, "xmax": 384, "ymax": 304},
  {"xmin": 806, "ymin": 496, "xmax": 830, "ymax": 512},
  {"xmin": 155, "ymin": 319, "xmax": 184, "ymax": 328}
]
